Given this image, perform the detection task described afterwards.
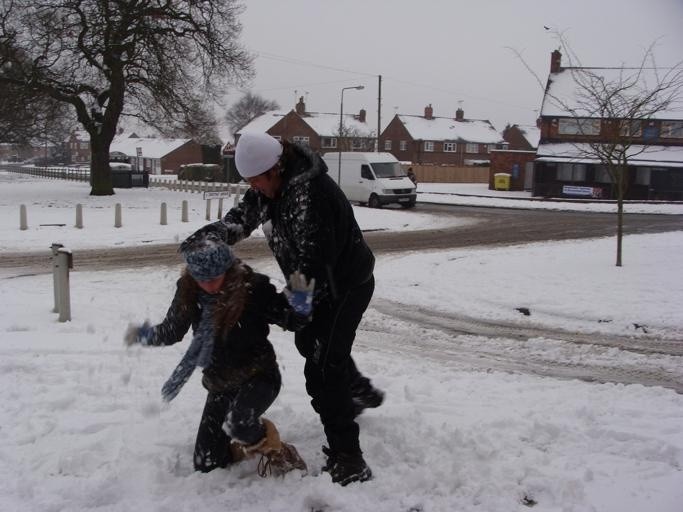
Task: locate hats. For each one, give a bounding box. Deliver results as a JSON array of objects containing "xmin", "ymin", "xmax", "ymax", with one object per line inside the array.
[
  {"xmin": 184, "ymin": 231, "xmax": 237, "ymax": 281},
  {"xmin": 233, "ymin": 129, "xmax": 285, "ymax": 178}
]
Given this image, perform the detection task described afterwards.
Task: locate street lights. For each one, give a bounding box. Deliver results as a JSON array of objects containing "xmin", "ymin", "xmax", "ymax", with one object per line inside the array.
[
  {"xmin": 336, "ymin": 83, "xmax": 371, "ymax": 201},
  {"xmin": 271, "ymin": 112, "xmax": 290, "ymax": 147},
  {"xmin": 43, "ymin": 116, "xmax": 69, "ymax": 169}
]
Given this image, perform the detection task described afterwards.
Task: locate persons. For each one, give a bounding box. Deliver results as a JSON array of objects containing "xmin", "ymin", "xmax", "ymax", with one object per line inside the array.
[
  {"xmin": 169, "ymin": 132, "xmax": 389, "ymax": 484},
  {"xmin": 118, "ymin": 230, "xmax": 318, "ymax": 476},
  {"xmin": 405, "ymin": 168, "xmax": 417, "ymax": 189}
]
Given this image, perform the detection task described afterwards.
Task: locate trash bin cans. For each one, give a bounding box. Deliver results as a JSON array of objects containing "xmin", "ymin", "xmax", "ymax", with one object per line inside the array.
[
  {"xmin": 178, "ymin": 162, "xmax": 222, "ymax": 182},
  {"xmin": 494, "ymin": 173, "xmax": 512, "ymax": 191}
]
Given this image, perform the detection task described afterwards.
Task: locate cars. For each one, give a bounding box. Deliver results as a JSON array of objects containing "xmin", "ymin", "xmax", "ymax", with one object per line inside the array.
[
  {"xmin": 23, "ymin": 155, "xmax": 60, "ymax": 168},
  {"xmin": 6, "ymin": 154, "xmax": 27, "ymax": 164}
]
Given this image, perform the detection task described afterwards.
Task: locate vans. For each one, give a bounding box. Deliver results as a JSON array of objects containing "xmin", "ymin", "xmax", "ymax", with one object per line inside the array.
[{"xmin": 321, "ymin": 152, "xmax": 418, "ymax": 211}]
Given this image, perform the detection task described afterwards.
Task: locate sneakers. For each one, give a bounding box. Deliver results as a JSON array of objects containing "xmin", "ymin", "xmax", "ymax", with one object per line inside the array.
[
  {"xmin": 319, "ymin": 443, "xmax": 372, "ymax": 486},
  {"xmin": 353, "ymin": 378, "xmax": 386, "ymax": 419}
]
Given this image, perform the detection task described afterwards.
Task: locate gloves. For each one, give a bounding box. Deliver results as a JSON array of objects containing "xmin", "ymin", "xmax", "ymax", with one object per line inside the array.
[
  {"xmin": 176, "ymin": 224, "xmax": 237, "ymax": 248},
  {"xmin": 283, "ymin": 270, "xmax": 317, "ymax": 315}
]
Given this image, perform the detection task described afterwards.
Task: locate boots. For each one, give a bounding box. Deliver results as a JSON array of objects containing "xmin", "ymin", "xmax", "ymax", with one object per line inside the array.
[
  {"xmin": 243, "ymin": 417, "xmax": 308, "ymax": 478},
  {"xmin": 229, "ymin": 438, "xmax": 256, "ymax": 462}
]
